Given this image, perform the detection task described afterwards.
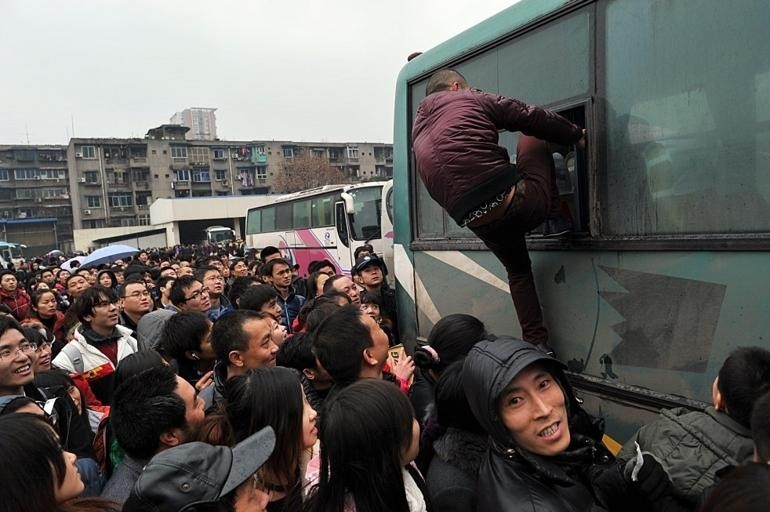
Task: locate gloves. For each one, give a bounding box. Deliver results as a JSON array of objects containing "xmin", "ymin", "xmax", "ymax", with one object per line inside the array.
[{"xmin": 631, "ymin": 454, "xmax": 673, "ymax": 504}]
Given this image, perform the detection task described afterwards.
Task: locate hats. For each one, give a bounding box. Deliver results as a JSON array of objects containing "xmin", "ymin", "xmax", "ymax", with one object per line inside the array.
[
  {"xmin": 354, "ymin": 253, "xmax": 381, "ymax": 273},
  {"xmin": 121, "ymin": 424, "xmax": 277, "ymax": 512}
]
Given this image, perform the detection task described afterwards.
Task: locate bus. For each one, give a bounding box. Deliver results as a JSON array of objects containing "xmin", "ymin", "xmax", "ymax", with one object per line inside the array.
[
  {"xmin": 381, "ymin": 179, "xmax": 394, "ymax": 290},
  {"xmin": 0, "ymin": 240, "xmax": 28, "ymax": 269},
  {"xmin": 202, "ymin": 225, "xmax": 237, "ymax": 249},
  {"xmin": 243, "ymin": 179, "xmax": 390, "ymax": 283},
  {"xmin": 391, "ymin": 1, "xmax": 768, "ymax": 461}
]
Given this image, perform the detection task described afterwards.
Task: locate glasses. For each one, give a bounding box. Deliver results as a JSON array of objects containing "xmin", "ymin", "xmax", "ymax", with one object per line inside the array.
[
  {"xmin": 87, "ymin": 300, "xmax": 120, "ymax": 311},
  {"xmin": 121, "ymin": 289, "xmax": 154, "ymax": 299},
  {"xmin": 201, "ymin": 275, "xmax": 225, "ymax": 281},
  {"xmin": 0, "ymin": 341, "xmax": 38, "ymax": 360},
  {"xmin": 182, "ymin": 286, "xmax": 211, "ymax": 304},
  {"xmin": 46, "ymin": 411, "xmax": 60, "ymax": 426}
]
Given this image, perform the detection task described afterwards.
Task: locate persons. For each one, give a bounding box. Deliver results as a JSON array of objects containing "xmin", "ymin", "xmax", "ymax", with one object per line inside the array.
[
  {"xmin": 748, "ymin": 394, "xmax": 770, "ymax": 465},
  {"xmin": 702, "ymin": 460, "xmax": 770, "ymax": 512},
  {"xmin": 615, "ymin": 344, "xmax": 770, "ymax": 511},
  {"xmin": 409, "ymin": 311, "xmax": 487, "ymax": 476},
  {"xmin": 459, "ymin": 334, "xmax": 679, "ymax": 511},
  {"xmin": 422, "ymin": 358, "xmax": 490, "ymax": 511},
  {"xmin": 408, "ymin": 66, "xmax": 587, "ymax": 364}
]
